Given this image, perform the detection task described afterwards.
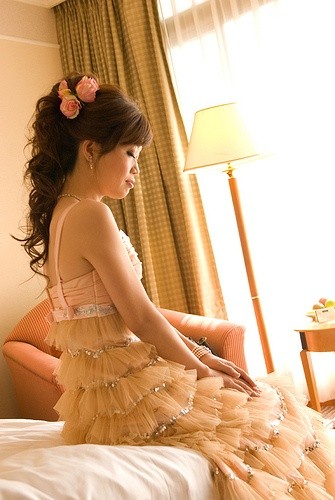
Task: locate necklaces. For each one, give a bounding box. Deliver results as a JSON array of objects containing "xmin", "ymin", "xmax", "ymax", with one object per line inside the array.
[{"xmin": 58, "ymin": 193, "xmax": 80, "ymax": 201}]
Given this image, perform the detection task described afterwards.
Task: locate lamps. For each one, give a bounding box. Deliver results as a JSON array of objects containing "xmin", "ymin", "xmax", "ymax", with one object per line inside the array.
[{"xmin": 183, "ymin": 103, "xmax": 276, "ymax": 375}]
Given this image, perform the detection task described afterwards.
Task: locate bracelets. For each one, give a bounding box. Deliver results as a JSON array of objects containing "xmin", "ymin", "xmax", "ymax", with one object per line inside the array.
[{"xmin": 191, "ymin": 344, "xmax": 212, "ymax": 359}]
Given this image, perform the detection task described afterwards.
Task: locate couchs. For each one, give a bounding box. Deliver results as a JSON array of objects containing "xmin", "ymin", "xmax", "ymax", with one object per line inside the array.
[{"xmin": 2, "ymin": 297, "xmax": 248, "ymax": 421}]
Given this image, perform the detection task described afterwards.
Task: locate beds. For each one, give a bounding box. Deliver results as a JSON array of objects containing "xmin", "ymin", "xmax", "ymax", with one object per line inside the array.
[{"xmin": 0, "ymin": 419, "xmax": 214, "ymax": 500}]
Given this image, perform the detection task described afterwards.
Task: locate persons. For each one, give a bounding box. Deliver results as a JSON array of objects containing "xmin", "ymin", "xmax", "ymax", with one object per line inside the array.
[{"xmin": 9, "ymin": 71, "xmax": 335, "ymax": 500}]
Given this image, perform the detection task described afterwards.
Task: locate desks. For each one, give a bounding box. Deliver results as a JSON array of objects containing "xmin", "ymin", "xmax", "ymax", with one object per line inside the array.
[{"xmin": 294, "ymin": 323, "xmax": 335, "ymax": 414}]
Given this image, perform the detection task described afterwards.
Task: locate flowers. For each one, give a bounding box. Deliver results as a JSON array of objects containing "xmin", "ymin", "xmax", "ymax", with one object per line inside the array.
[{"xmin": 58, "ymin": 75, "xmax": 100, "ymax": 119}]
